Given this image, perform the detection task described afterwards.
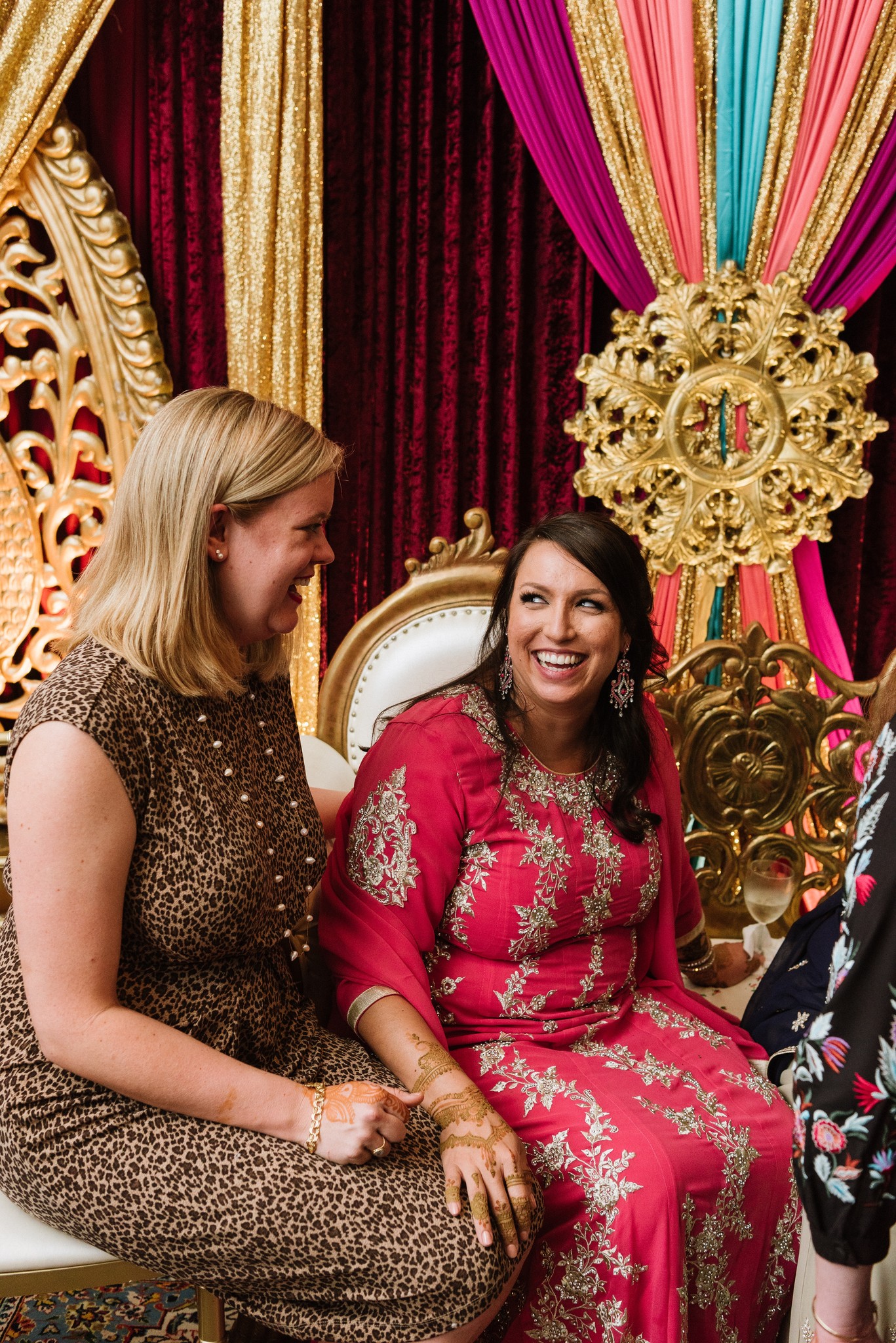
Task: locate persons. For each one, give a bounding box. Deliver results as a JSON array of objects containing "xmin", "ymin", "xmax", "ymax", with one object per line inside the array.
[
  {"xmin": 786, "ymin": 710, "xmax": 895, "ymax": 1343},
  {"xmin": 319, "ymin": 509, "xmax": 806, "ymax": 1343},
  {"xmin": 0, "ymin": 389, "xmax": 549, "ymax": 1343}
]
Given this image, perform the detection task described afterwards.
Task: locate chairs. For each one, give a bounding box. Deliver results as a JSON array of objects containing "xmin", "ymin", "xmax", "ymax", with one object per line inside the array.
[
  {"xmin": 641, "ymin": 618, "xmax": 896, "ymax": 1022},
  {"xmin": 317, "ymin": 509, "xmax": 508, "ymax": 779},
  {"xmin": 1, "ymin": 1189, "xmax": 229, "ymax": 1343}
]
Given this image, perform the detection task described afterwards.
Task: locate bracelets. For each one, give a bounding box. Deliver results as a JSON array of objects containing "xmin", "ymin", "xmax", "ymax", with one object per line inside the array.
[
  {"xmin": 302, "ymin": 1081, "xmax": 326, "ymax": 1155},
  {"xmin": 678, "ymin": 944, "xmax": 716, "ymax": 973},
  {"xmin": 811, "ymin": 1292, "xmax": 880, "ymax": 1343}
]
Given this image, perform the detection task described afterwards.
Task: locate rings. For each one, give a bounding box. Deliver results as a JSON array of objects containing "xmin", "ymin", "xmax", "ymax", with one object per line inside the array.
[{"xmin": 371, "ymin": 1134, "xmax": 386, "ymax": 1157}]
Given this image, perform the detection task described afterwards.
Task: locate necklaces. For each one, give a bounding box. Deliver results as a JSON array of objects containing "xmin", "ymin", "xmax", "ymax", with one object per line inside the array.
[{"xmin": 187, "ymin": 666, "xmax": 317, "ymax": 961}]
